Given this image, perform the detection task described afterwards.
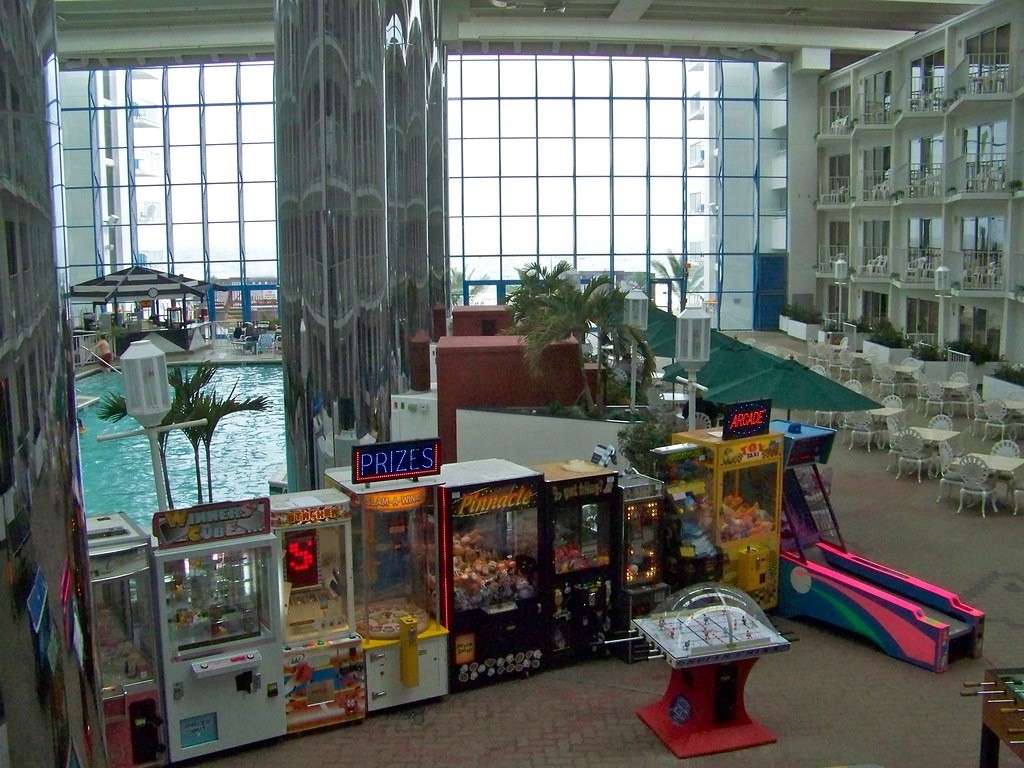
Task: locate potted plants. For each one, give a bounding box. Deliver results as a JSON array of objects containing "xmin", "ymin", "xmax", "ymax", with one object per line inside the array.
[
  {"xmin": 946, "ymin": 187, "xmax": 956, "ymax": 197},
  {"xmin": 779, "ymin": 294, "xmax": 1024, "ymax": 416},
  {"xmin": 850, "ymin": 197, "xmax": 856, "ymax": 203},
  {"xmin": 889, "ymin": 273, "xmax": 900, "ymax": 281},
  {"xmin": 1007, "ymin": 180, "xmax": 1021, "ymax": 192},
  {"xmin": 895, "ymin": 110, "xmax": 901, "ymax": 121},
  {"xmin": 897, "ymin": 189, "xmax": 903, "ymax": 199},
  {"xmin": 956, "ymin": 87, "xmax": 964, "ymax": 99},
  {"xmin": 950, "ymin": 282, "xmax": 961, "ymax": 295},
  {"xmin": 851, "ymin": 119, "xmax": 859, "ymax": 129},
  {"xmin": 813, "ymin": 199, "xmax": 819, "ymax": 209}
]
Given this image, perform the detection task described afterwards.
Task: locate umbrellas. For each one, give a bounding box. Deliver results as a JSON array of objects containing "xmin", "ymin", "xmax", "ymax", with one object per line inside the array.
[
  {"xmin": 637, "ymin": 306, "xmax": 745, "ymax": 410},
  {"xmin": 660, "ymin": 341, "xmax": 786, "ymax": 388},
  {"xmin": 699, "ymin": 359, "xmax": 885, "ymax": 422}
]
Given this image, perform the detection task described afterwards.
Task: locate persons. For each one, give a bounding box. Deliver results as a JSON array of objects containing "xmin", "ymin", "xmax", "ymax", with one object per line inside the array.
[
  {"xmin": 683, "ymin": 640, "xmax": 690, "ymax": 650},
  {"xmin": 704, "ymin": 615, "xmax": 710, "ymax": 625},
  {"xmin": 234, "ymin": 320, "xmax": 277, "ymax": 354},
  {"xmin": 91, "ymin": 335, "xmax": 112, "ymax": 373},
  {"xmin": 704, "ymin": 628, "xmax": 710, "ymax": 640},
  {"xmin": 742, "ymin": 616, "xmax": 746, "ymax": 624},
  {"xmin": 670, "ymin": 628, "xmax": 675, "ymax": 639},
  {"xmin": 659, "ymin": 617, "xmax": 664, "ymax": 630},
  {"xmin": 734, "ymin": 619, "xmax": 737, "ymax": 629},
  {"xmin": 723, "ymin": 627, "xmax": 728, "ymax": 634}
]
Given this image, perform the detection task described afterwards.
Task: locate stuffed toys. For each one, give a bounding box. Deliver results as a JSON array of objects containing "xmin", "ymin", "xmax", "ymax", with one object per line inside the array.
[
  {"xmin": 555, "ymin": 548, "xmax": 608, "ymax": 572},
  {"xmin": 682, "ymin": 494, "xmax": 774, "ymax": 539},
  {"xmin": 423, "ymin": 531, "xmax": 534, "ymax": 609}
]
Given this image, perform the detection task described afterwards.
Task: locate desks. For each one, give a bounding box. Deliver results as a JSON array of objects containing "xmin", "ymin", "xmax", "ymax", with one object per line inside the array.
[
  {"xmin": 893, "ymin": 426, "xmax": 961, "ymax": 475},
  {"xmin": 978, "ymin": 400, "xmax": 1024, "ymax": 434},
  {"xmin": 860, "ymin": 408, "xmax": 905, "ymax": 447},
  {"xmin": 878, "ymin": 363, "xmax": 915, "ymax": 393},
  {"xmin": 921, "ymin": 382, "xmax": 969, "ymax": 411},
  {"xmin": 952, "ymin": 453, "xmax": 1024, "ymax": 504},
  {"xmin": 830, "ymin": 345, "xmax": 849, "ymax": 351},
  {"xmin": 853, "ymin": 351, "xmax": 864, "ymax": 359}
]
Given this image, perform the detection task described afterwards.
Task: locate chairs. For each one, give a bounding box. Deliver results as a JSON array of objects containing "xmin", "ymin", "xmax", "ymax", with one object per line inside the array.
[
  {"xmin": 816, "ymin": 69, "xmax": 1012, "ymax": 291},
  {"xmin": 226, "ymin": 322, "xmax": 282, "ymax": 360},
  {"xmin": 744, "ymin": 338, "xmax": 1024, "ymax": 518}
]
